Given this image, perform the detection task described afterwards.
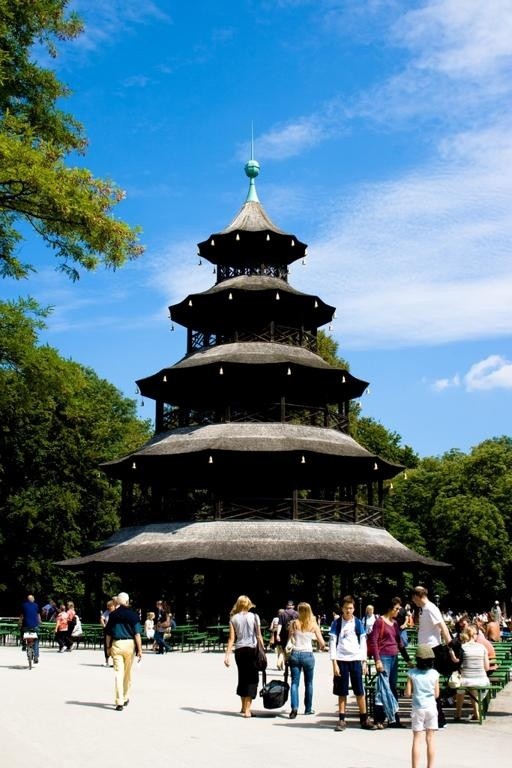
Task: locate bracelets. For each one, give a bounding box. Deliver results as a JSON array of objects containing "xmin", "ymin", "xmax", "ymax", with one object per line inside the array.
[{"xmin": 106, "ymin": 645, "xmax": 110, "ymax": 648}]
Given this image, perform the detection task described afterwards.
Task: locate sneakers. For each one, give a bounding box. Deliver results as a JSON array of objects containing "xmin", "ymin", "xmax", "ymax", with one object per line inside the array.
[
  {"xmin": 454, "ymin": 716, "xmax": 461, "ymax": 721},
  {"xmin": 469, "ymin": 714, "xmax": 480, "ymax": 720},
  {"xmin": 151, "ymin": 645, "xmax": 171, "ymax": 654},
  {"xmin": 386, "ymin": 722, "xmax": 406, "ymax": 728},
  {"xmin": 361, "ymin": 719, "xmax": 384, "ymax": 729},
  {"xmin": 290, "ymin": 710, "xmax": 297, "ymax": 718},
  {"xmin": 304, "ymin": 709, "xmax": 315, "ymax": 715},
  {"xmin": 334, "ymin": 720, "xmax": 347, "ymax": 731},
  {"xmin": 101, "ymin": 663, "xmax": 110, "ymax": 668}
]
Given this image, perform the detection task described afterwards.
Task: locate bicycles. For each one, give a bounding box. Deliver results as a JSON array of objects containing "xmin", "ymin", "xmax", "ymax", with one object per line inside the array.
[{"xmin": 19, "ymin": 625, "xmax": 41, "ymax": 670}]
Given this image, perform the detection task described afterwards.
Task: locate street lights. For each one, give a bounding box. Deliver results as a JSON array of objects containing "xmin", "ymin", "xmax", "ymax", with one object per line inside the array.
[
  {"xmin": 358, "ymin": 597, "xmax": 362, "ymax": 619},
  {"xmin": 433, "ymin": 594, "xmax": 440, "ymax": 607}
]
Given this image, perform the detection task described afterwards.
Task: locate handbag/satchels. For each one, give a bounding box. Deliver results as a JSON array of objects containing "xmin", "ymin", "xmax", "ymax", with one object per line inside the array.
[
  {"xmin": 366, "ymin": 638, "xmax": 381, "ymax": 656},
  {"xmin": 285, "ymin": 636, "xmax": 295, "ymax": 660},
  {"xmin": 251, "ymin": 646, "xmax": 267, "ymax": 671},
  {"xmin": 447, "ymin": 670, "xmax": 462, "ymax": 689},
  {"xmin": 259, "ymin": 679, "xmax": 290, "ymax": 708},
  {"xmin": 157, "ymin": 625, "xmax": 172, "ymax": 639},
  {"xmin": 50, "ymin": 609, "xmax": 61, "ymax": 622}
]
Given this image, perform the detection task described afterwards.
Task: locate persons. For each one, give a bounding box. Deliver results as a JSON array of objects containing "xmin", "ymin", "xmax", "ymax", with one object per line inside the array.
[
  {"xmin": 328, "ymin": 595, "xmax": 379, "ymax": 729},
  {"xmin": 41, "ymin": 596, "xmax": 176, "ymax": 653},
  {"xmin": 372, "ymin": 596, "xmax": 414, "ymax": 728},
  {"xmin": 404, "ymin": 642, "xmax": 441, "ymax": 767},
  {"xmin": 104, "ymin": 591, "xmax": 143, "ymax": 710},
  {"xmin": 411, "ymin": 584, "xmax": 460, "ymax": 727},
  {"xmin": 287, "ymin": 601, "xmax": 326, "ymax": 718},
  {"xmin": 267, "ymin": 600, "xmax": 511, "ymax": 670},
  {"xmin": 224, "ymin": 594, "xmax": 265, "ymax": 717},
  {"xmin": 453, "ymin": 623, "xmax": 491, "ymax": 720},
  {"xmin": 19, "ymin": 594, "xmax": 42, "ymax": 664}
]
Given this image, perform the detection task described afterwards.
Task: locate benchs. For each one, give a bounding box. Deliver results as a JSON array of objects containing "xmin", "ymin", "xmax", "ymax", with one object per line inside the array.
[{"xmin": 348, "ymin": 623, "xmax": 512, "ymax": 725}]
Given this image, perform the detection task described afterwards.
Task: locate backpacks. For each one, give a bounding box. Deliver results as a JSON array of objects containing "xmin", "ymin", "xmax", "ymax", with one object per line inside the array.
[
  {"xmin": 280, "ymin": 616, "xmax": 294, "ymax": 642},
  {"xmin": 68, "ymin": 614, "xmax": 82, "ymax": 642}
]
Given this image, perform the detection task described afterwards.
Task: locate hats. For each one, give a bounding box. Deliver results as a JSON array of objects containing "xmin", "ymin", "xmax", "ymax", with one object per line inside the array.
[
  {"xmin": 494, "ymin": 600, "xmax": 500, "ymax": 605},
  {"xmin": 415, "ymin": 644, "xmax": 436, "ymax": 660},
  {"xmin": 287, "ymin": 600, "xmax": 295, "ymax": 606},
  {"xmin": 112, "ymin": 592, "xmax": 130, "ymax": 604}
]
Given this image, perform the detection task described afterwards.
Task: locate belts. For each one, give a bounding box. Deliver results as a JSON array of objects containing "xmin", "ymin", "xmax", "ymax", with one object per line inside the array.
[{"xmin": 113, "ymin": 637, "xmax": 134, "ymax": 641}]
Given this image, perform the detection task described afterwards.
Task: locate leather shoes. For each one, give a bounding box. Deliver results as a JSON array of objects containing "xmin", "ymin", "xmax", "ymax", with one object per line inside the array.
[
  {"xmin": 239, "ymin": 710, "xmax": 256, "ymax": 718},
  {"xmin": 116, "ymin": 697, "xmax": 130, "ymax": 711}
]
